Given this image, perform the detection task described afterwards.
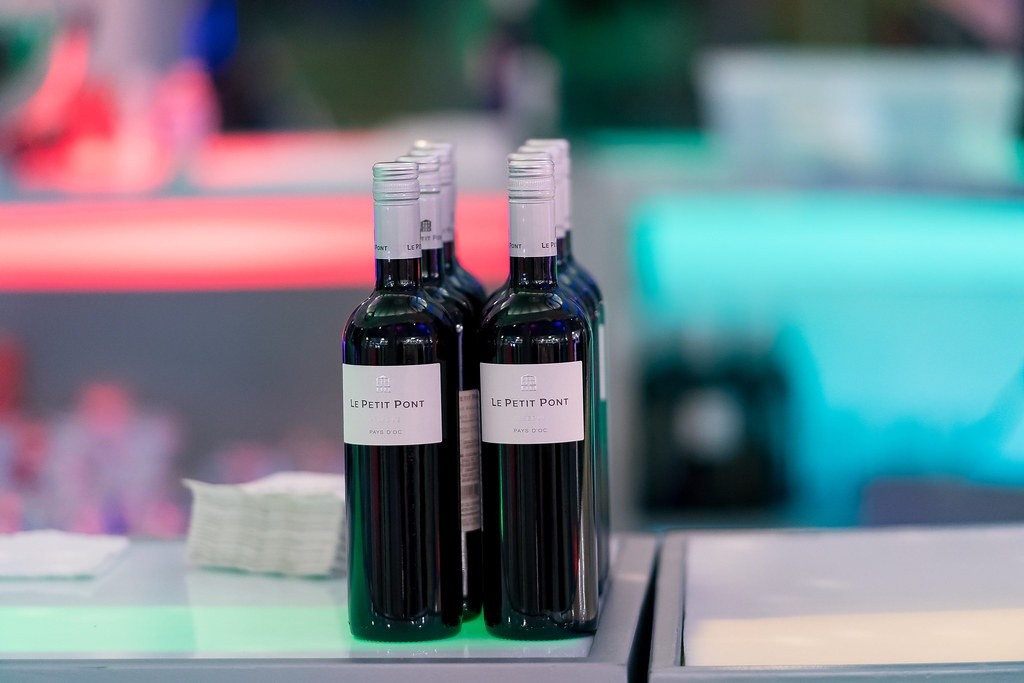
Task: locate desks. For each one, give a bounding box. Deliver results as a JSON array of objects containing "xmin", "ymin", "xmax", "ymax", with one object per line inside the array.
[
  {"xmin": 646, "ymin": 521, "xmax": 1024, "ymax": 683},
  {"xmin": 1, "ymin": 531, "xmax": 656, "ymax": 681}
]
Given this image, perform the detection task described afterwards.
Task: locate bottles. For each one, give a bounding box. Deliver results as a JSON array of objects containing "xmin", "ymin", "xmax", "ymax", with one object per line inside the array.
[
  {"xmin": 395, "ymin": 140, "xmax": 487, "ymax": 625},
  {"xmin": 518, "ymin": 136, "xmax": 610, "ymax": 601},
  {"xmin": 340, "ymin": 160, "xmax": 463, "ymax": 643},
  {"xmin": 476, "ymin": 153, "xmax": 598, "ymax": 643}
]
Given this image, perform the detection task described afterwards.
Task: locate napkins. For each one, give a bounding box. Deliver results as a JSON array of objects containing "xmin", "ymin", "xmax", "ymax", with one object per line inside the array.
[
  {"xmin": 182, "ymin": 472, "xmax": 346, "ymax": 579},
  {"xmin": 3, "ymin": 528, "xmax": 131, "ymax": 580}
]
